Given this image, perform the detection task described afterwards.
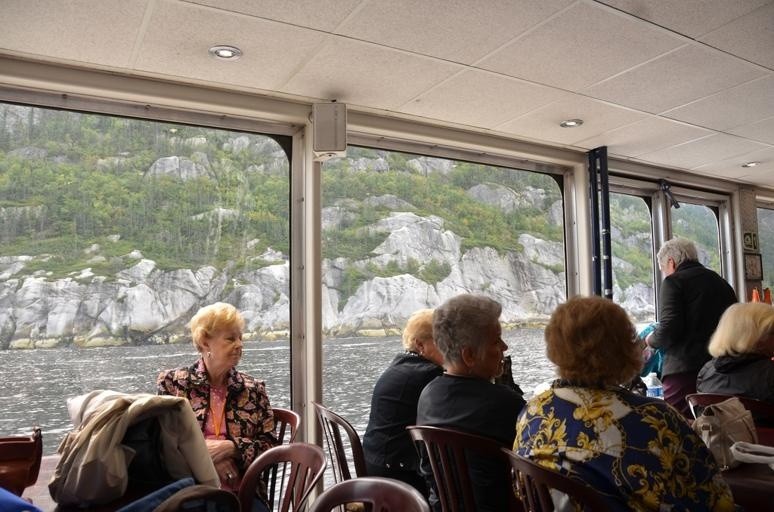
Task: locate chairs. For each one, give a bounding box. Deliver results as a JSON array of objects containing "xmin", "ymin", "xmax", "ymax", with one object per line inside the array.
[
  {"xmin": 311, "ymin": 402, "xmax": 371, "ymax": 511},
  {"xmin": 307, "ymin": 477, "xmax": 429, "ymax": 512},
  {"xmin": 262, "ymin": 408, "xmax": 301, "ymax": 511},
  {"xmin": 685, "ymin": 392, "xmax": 768, "ymax": 429},
  {"xmin": 155, "ymin": 486, "xmax": 241, "ymax": 512},
  {"xmin": 237, "ymin": 443, "xmax": 328, "ymax": 511},
  {"xmin": 406, "ymin": 426, "xmax": 501, "ymax": 511},
  {"xmin": 500, "ymin": 447, "xmax": 628, "ymax": 512}
]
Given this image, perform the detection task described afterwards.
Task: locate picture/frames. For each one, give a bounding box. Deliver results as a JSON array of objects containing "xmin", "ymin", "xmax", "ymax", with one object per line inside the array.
[{"xmin": 743, "ymin": 252, "xmax": 764, "ymax": 283}]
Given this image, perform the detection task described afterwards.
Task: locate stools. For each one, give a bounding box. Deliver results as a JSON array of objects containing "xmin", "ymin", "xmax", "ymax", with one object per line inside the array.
[{"xmin": 1, "ymin": 426, "xmax": 43, "ymax": 498}]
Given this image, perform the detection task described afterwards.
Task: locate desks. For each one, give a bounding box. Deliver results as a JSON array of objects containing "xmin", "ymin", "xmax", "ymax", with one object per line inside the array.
[{"xmin": 719, "ymin": 430, "xmax": 773, "ymax": 511}]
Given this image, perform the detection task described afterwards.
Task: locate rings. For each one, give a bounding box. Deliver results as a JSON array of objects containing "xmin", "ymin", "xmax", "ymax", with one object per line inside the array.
[{"xmin": 225, "ymin": 471, "xmax": 233, "ymax": 483}]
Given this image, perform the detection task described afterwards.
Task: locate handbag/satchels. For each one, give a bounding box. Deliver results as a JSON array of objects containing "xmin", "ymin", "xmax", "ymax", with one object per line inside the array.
[{"xmin": 691, "ymin": 397, "xmax": 757, "ymax": 468}]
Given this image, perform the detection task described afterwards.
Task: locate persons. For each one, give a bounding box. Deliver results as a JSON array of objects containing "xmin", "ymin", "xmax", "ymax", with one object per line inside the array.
[
  {"xmin": 362, "ymin": 309, "xmax": 446, "ymax": 512},
  {"xmin": 510, "ymin": 294, "xmax": 734, "ymax": 511},
  {"xmin": 696, "ymin": 302, "xmax": 774, "ymax": 427},
  {"xmin": 416, "ymin": 294, "xmax": 527, "ymax": 511},
  {"xmin": 156, "ymin": 301, "xmax": 277, "ymax": 512},
  {"xmin": 644, "ymin": 237, "xmax": 739, "ymax": 419}
]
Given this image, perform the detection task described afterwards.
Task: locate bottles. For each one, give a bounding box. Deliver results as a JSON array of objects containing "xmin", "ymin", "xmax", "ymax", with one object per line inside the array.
[
  {"xmin": 751, "ymin": 286, "xmax": 760, "ymax": 302},
  {"xmin": 762, "ymin": 287, "xmax": 772, "ymax": 305},
  {"xmin": 645, "ymin": 372, "xmax": 665, "ymax": 400}
]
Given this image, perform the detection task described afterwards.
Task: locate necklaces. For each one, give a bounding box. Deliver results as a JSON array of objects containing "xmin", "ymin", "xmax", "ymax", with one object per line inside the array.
[{"xmin": 210, "ymin": 392, "xmax": 229, "ymax": 436}]
